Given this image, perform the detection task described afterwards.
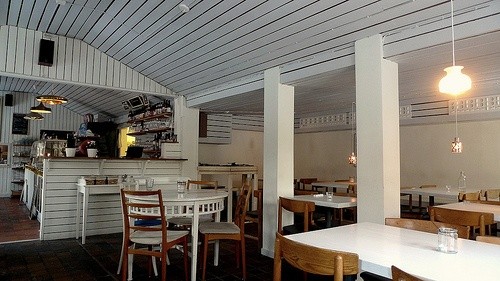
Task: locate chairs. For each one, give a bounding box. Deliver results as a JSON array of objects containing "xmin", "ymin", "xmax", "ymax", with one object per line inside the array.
[
  {"xmin": 300, "ymin": 178, "xmax": 322, "ymax": 194},
  {"xmin": 385, "ymin": 185, "xmax": 500, "ymax": 245},
  {"xmin": 117, "ymin": 178, "xmax": 263, "ymax": 281},
  {"xmin": 273, "ymin": 231, "xmax": 359, "ymax": 281},
  {"xmin": 278, "ymin": 196, "xmax": 323, "ymax": 281}
]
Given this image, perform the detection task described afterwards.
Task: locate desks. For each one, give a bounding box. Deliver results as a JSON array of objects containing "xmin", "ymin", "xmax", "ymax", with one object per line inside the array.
[
  {"xmin": 428, "ymin": 201, "xmax": 500, "ymax": 236},
  {"xmin": 76, "ymin": 182, "xmax": 137, "ymax": 244},
  {"xmin": 199, "ymin": 166, "xmax": 259, "ymax": 226},
  {"xmin": 400, "ymin": 186, "xmax": 482, "ymax": 207},
  {"xmin": 124, "ymin": 189, "xmax": 228, "ymax": 281},
  {"xmin": 311, "ymin": 180, "xmax": 356, "ymax": 221},
  {"xmin": 284, "ymin": 222, "xmax": 500, "ymax": 281},
  {"xmin": 290, "ymin": 193, "xmax": 357, "ymax": 228}
]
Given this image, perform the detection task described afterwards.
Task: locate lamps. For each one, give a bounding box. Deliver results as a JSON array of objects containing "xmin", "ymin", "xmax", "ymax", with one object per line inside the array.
[
  {"xmin": 438, "ymin": 0, "xmax": 472, "ymax": 94},
  {"xmin": 34, "ymin": 88, "xmax": 68, "ymax": 105},
  {"xmin": 30, "ymin": 101, "xmax": 52, "ymax": 114},
  {"xmin": 348, "ymin": 100, "xmax": 357, "ymax": 167},
  {"xmin": 450, "ymin": 95, "xmax": 463, "ymax": 154},
  {"xmin": 23, "ymin": 111, "xmax": 45, "ymax": 121}
]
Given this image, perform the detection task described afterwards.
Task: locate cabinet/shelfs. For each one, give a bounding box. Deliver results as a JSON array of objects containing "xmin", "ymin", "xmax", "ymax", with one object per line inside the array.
[
  {"xmin": 125, "ymin": 112, "xmax": 174, "ymax": 154},
  {"xmin": 11, "ymin": 144, "xmax": 31, "ymax": 196}
]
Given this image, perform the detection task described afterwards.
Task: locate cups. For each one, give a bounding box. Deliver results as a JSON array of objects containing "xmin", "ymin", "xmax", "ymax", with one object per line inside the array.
[
  {"xmin": 437, "ymin": 228, "xmax": 458, "ymax": 254},
  {"xmin": 87, "ymin": 149, "xmax": 97, "ymax": 157},
  {"xmin": 146, "ymin": 178, "xmax": 154, "ymax": 189},
  {"xmin": 65, "ymin": 148, "xmax": 76, "ymax": 157},
  {"xmin": 326, "ymin": 192, "xmax": 333, "ymax": 201},
  {"xmin": 349, "ymin": 176, "xmax": 355, "ymax": 183},
  {"xmin": 177, "ymin": 181, "xmax": 186, "ymax": 193}
]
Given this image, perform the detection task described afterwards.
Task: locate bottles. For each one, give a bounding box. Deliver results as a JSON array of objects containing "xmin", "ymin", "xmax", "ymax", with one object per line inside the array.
[{"xmin": 458, "ymin": 171, "xmax": 466, "ymax": 193}]
